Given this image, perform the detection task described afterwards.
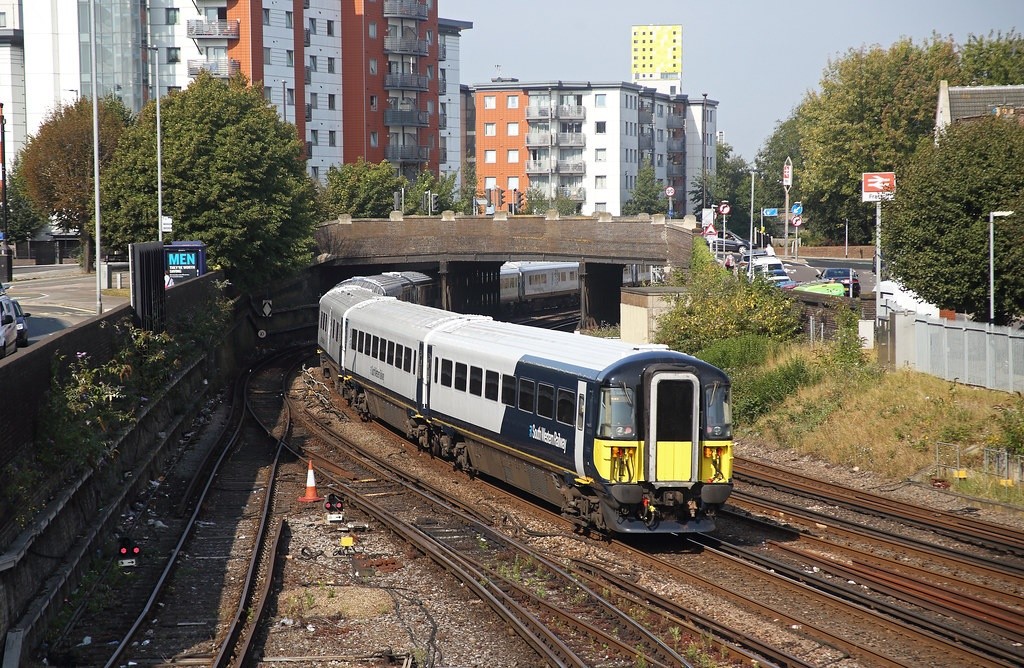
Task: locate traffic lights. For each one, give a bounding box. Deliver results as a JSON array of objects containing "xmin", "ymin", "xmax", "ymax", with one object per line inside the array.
[
  {"xmin": 392, "ymin": 192, "xmax": 400, "ymax": 211},
  {"xmin": 322, "ymin": 493, "xmax": 343, "ymax": 513},
  {"xmin": 420, "ymin": 195, "xmax": 428, "ymax": 209},
  {"xmin": 498, "ymin": 189, "xmax": 506, "ymax": 207},
  {"xmin": 431, "ymin": 194, "xmax": 439, "ymax": 212},
  {"xmin": 516, "ymin": 192, "xmax": 524, "ymax": 210}
]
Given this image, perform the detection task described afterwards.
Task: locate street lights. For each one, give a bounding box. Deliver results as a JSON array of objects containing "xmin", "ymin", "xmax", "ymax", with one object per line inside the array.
[
  {"xmin": 701, "ymin": 92, "xmax": 709, "ymax": 239},
  {"xmin": 989, "ymin": 209, "xmax": 1016, "ymax": 326},
  {"xmin": 749, "ymin": 168, "xmax": 762, "ymax": 282},
  {"xmin": 139, "ymin": 45, "xmax": 163, "ymax": 244},
  {"xmin": 281, "ymin": 79, "xmax": 288, "ymax": 122},
  {"xmin": 547, "ymin": 87, "xmax": 554, "ymax": 210}
]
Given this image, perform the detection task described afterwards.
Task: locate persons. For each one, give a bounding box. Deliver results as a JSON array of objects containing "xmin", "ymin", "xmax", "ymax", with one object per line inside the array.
[{"xmin": 164, "ymin": 271, "xmax": 174, "ymax": 289}]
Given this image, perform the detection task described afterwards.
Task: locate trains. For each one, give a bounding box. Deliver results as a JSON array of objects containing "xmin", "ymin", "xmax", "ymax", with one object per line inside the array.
[{"xmin": 316, "ymin": 261, "xmax": 734, "ymax": 536}]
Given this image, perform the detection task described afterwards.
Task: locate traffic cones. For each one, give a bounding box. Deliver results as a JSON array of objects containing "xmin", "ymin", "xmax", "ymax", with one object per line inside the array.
[{"xmin": 296, "ymin": 460, "xmax": 325, "ymax": 503}]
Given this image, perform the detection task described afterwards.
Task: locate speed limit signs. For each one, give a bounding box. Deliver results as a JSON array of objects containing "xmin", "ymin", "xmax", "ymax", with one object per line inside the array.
[{"xmin": 666, "ymin": 187, "xmax": 675, "ymax": 197}]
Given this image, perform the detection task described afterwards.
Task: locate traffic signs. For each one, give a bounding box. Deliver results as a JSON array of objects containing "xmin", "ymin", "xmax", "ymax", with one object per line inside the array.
[{"xmin": 763, "ymin": 208, "xmax": 779, "ymax": 217}]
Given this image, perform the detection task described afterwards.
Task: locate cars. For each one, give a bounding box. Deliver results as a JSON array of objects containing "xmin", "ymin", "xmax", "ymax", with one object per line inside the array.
[
  {"xmin": 736, "ymin": 250, "xmax": 845, "ymax": 309},
  {"xmin": 0, "ymin": 281, "xmax": 32, "ymax": 361},
  {"xmin": 815, "ymin": 267, "xmax": 861, "ymax": 298},
  {"xmin": 706, "ymin": 229, "xmax": 758, "ymax": 255}
]
